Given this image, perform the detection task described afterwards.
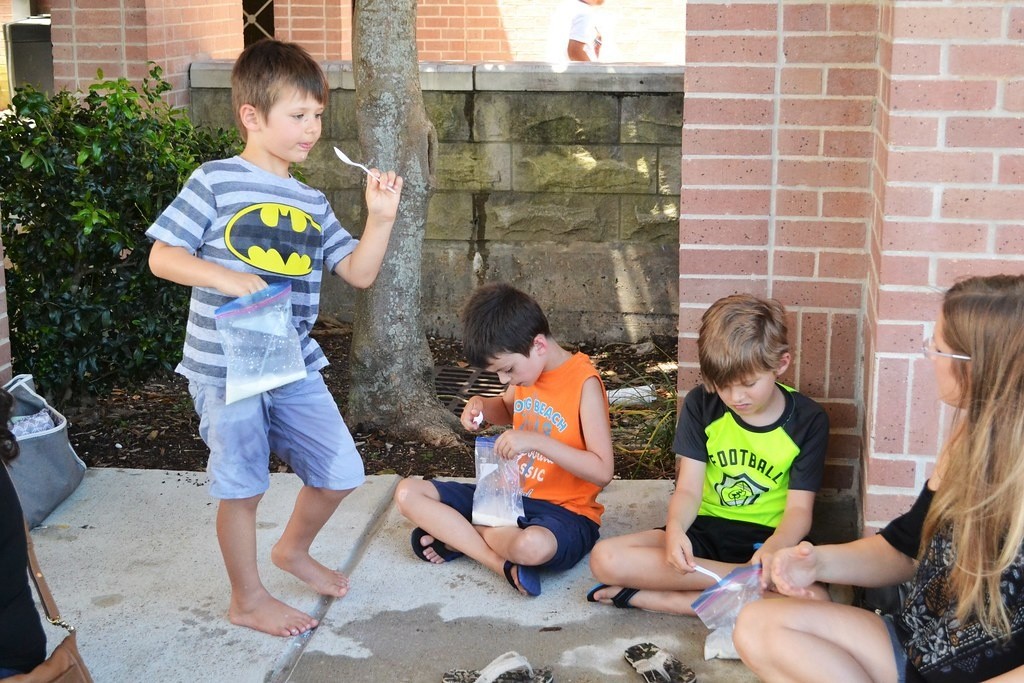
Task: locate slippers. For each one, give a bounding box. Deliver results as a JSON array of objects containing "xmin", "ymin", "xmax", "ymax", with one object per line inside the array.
[
  {"xmin": 624, "ymin": 643, "xmax": 696, "ymax": 683},
  {"xmin": 411, "ymin": 525, "xmax": 464, "ymax": 565},
  {"xmin": 504, "ymin": 559, "xmax": 541, "ymax": 597},
  {"xmin": 442, "ymin": 650, "xmax": 553, "ymax": 683},
  {"xmin": 587, "ymin": 586, "xmax": 639, "ymax": 608}
]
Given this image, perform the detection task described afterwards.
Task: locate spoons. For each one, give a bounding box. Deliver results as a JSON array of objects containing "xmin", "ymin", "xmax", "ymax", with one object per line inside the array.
[
  {"xmin": 334, "ymin": 147, "xmax": 396, "ymax": 195},
  {"xmin": 474, "ymin": 409, "xmax": 483, "ymax": 426},
  {"xmin": 693, "ymin": 566, "xmax": 743, "ymax": 593}
]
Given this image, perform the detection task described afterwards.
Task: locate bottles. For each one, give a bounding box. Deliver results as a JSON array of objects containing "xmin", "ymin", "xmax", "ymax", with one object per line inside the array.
[{"xmin": 605, "ymin": 384, "xmax": 657, "ymax": 406}]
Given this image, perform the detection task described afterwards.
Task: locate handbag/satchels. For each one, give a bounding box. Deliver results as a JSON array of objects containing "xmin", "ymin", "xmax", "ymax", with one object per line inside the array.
[
  {"xmin": 0, "ymin": 630, "xmax": 93, "ymax": 682},
  {"xmin": 2, "ymin": 373, "xmax": 87, "ymax": 530}
]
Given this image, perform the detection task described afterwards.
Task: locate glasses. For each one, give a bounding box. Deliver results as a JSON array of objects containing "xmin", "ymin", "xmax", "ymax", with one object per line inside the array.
[{"xmin": 921, "ymin": 339, "xmax": 971, "ymax": 361}]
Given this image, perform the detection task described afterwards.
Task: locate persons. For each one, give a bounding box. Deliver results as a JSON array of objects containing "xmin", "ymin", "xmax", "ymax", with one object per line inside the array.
[
  {"xmin": 734, "ymin": 274, "xmax": 1024, "ymax": 683},
  {"xmin": 567, "ymin": 0, "xmax": 607, "ymax": 61},
  {"xmin": 588, "ymin": 293, "xmax": 831, "ymax": 617},
  {"xmin": 0, "ymin": 387, "xmax": 47, "ymax": 683},
  {"xmin": 145, "ymin": 39, "xmax": 404, "ymax": 638},
  {"xmin": 394, "ymin": 283, "xmax": 615, "ymax": 598}
]
